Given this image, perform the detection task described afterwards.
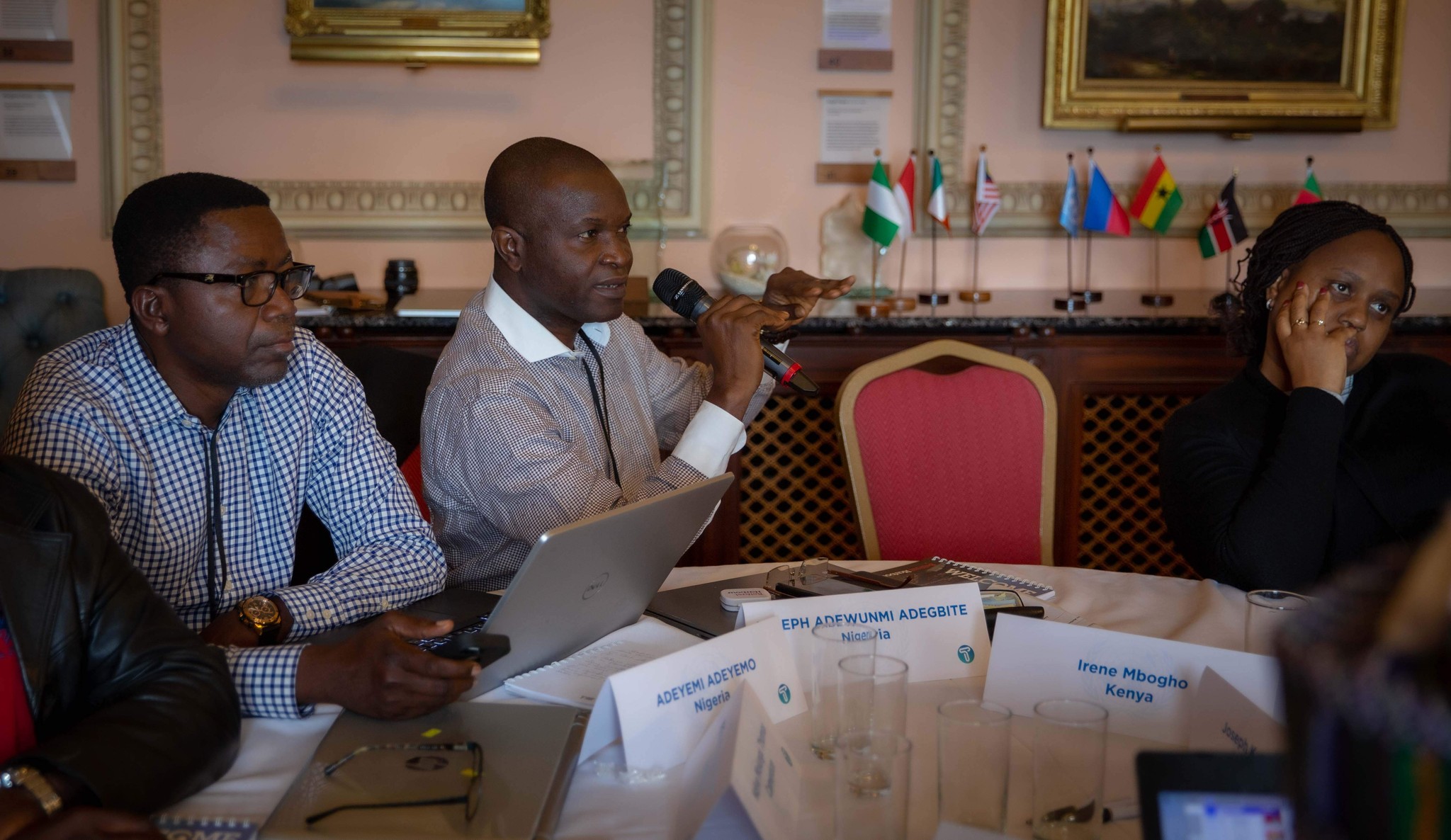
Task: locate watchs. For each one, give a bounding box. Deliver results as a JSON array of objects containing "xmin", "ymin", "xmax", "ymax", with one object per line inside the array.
[{"xmin": 234, "ymin": 596, "xmax": 282, "ymax": 646}]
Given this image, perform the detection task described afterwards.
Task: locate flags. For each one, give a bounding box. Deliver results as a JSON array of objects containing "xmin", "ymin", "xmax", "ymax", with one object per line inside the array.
[
  {"xmin": 860, "ymin": 160, "xmax": 903, "ymax": 248},
  {"xmin": 1197, "ymin": 176, "xmax": 1249, "ymax": 259},
  {"xmin": 1082, "ymin": 165, "xmax": 1131, "ymax": 237},
  {"xmin": 927, "ymin": 159, "xmax": 950, "ymax": 230},
  {"xmin": 892, "ymin": 155, "xmax": 916, "ymax": 243},
  {"xmin": 1059, "ymin": 166, "xmax": 1082, "ymax": 240},
  {"xmin": 1292, "ymin": 171, "xmax": 1323, "ymax": 205},
  {"xmin": 971, "ymin": 154, "xmax": 1001, "ymax": 238},
  {"xmin": 1130, "ymin": 155, "xmax": 1183, "ymax": 237}
]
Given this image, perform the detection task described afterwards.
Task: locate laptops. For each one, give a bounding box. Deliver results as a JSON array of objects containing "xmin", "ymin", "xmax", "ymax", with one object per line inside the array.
[{"xmin": 309, "ymin": 472, "xmax": 736, "ymax": 703}]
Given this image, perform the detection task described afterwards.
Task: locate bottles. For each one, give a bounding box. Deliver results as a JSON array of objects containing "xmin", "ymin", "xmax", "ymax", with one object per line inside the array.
[{"xmin": 385, "ymin": 259, "xmax": 421, "ymax": 309}]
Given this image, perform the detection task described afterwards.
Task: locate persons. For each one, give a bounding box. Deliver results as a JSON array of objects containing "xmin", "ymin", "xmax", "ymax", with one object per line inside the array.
[
  {"xmin": 0, "ymin": 454, "xmax": 242, "ymax": 840},
  {"xmin": 1158, "ymin": 199, "xmax": 1451, "ymax": 595},
  {"xmin": 418, "ymin": 135, "xmax": 857, "ymax": 582},
  {"xmin": 1, "ymin": 170, "xmax": 482, "ymax": 722}
]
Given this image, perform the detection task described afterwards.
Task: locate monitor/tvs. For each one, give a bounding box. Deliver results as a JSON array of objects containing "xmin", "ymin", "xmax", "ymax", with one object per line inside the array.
[{"xmin": 1135, "ymin": 752, "xmax": 1295, "ymax": 840}]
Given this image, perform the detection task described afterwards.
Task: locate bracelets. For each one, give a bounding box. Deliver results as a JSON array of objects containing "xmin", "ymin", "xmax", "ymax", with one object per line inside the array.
[{"xmin": 4, "ymin": 765, "xmax": 62, "ymax": 817}]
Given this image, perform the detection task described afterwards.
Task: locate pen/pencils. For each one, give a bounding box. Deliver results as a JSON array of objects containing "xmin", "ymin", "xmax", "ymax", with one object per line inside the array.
[
  {"xmin": 764, "ymin": 586, "xmax": 797, "ymax": 599},
  {"xmin": 1039, "ymin": 797, "xmax": 1142, "ymax": 824}
]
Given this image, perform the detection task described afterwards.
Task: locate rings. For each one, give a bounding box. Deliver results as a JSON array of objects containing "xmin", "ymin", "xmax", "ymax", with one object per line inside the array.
[
  {"xmin": 1309, "ymin": 319, "xmax": 1326, "ymax": 327},
  {"xmin": 1292, "ymin": 317, "xmax": 1309, "ymax": 328}
]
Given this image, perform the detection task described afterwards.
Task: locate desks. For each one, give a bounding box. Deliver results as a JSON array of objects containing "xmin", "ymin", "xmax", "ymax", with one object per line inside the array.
[{"xmin": 146, "ymin": 562, "xmax": 1324, "ymax": 839}]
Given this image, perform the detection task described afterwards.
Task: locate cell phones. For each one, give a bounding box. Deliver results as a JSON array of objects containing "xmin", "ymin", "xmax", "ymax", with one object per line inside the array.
[{"xmin": 426, "ymin": 631, "xmax": 509, "ymax": 670}]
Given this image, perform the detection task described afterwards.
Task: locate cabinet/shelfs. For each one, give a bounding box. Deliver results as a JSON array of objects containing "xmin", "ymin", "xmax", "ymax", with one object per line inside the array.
[{"xmin": 302, "ymin": 320, "xmax": 1449, "ymax": 584}]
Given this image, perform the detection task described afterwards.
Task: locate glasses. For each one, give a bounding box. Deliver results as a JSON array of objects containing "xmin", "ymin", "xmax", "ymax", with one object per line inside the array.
[{"xmin": 150, "ymin": 262, "xmax": 316, "ymax": 307}]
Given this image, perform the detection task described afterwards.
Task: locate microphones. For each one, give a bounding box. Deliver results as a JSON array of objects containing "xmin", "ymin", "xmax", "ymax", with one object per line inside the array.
[{"xmin": 653, "ymin": 268, "xmax": 819, "ymax": 393}]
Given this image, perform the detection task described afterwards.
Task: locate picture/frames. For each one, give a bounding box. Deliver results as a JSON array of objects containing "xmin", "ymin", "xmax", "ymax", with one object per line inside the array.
[
  {"xmin": 286, "ymin": 0, "xmax": 551, "ymax": 65},
  {"xmin": 1041, "ymin": 0, "xmax": 1403, "ymax": 135}
]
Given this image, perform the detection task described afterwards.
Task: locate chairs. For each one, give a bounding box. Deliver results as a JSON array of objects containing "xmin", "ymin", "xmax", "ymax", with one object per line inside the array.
[{"xmin": 832, "ymin": 341, "xmax": 1060, "ymax": 564}]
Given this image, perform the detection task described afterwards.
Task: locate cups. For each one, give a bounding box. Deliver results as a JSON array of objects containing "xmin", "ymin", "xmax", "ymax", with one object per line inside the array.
[
  {"xmin": 1031, "ymin": 698, "xmax": 1109, "ymax": 840},
  {"xmin": 936, "ymin": 699, "xmax": 1014, "ymax": 834},
  {"xmin": 833, "ymin": 728, "xmax": 913, "ymax": 840},
  {"xmin": 712, "ymin": 225, "xmax": 788, "ymax": 297},
  {"xmin": 1244, "ymin": 590, "xmax": 1310, "ymax": 655},
  {"xmin": 839, "ymin": 653, "xmax": 909, "ymax": 734},
  {"xmin": 810, "ymin": 622, "xmax": 876, "ymax": 758}
]
